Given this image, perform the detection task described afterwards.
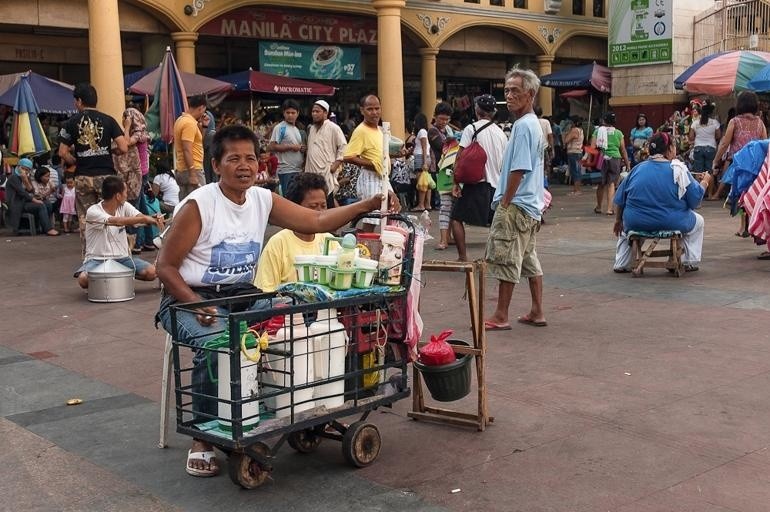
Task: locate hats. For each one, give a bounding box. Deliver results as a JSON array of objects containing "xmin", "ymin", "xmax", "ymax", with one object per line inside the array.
[
  {"xmin": 16, "ymin": 158, "xmax": 33, "ymax": 170},
  {"xmin": 474, "ymin": 95, "xmax": 497, "ymax": 111},
  {"xmin": 315, "ymin": 99, "xmax": 329, "ymax": 112}
]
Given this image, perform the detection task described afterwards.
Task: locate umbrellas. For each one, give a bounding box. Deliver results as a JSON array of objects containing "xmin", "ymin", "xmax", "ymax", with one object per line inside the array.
[
  {"xmin": 673, "ymin": 46, "xmax": 769, "ymax": 97},
  {"xmin": 143, "ymin": 45, "xmax": 190, "ymax": 145}
]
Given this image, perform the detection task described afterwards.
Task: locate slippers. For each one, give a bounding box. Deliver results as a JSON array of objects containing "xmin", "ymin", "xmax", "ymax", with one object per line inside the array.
[
  {"xmin": 606, "ymin": 209, "xmax": 616, "ymax": 215},
  {"xmin": 410, "ymin": 207, "xmax": 425, "ymax": 212},
  {"xmin": 614, "ymin": 268, "xmax": 632, "ymax": 273},
  {"xmin": 518, "ymin": 314, "xmax": 547, "ymax": 326},
  {"xmin": 471, "ymin": 322, "xmax": 512, "ymax": 331},
  {"xmin": 684, "ymin": 263, "xmax": 698, "ymax": 272},
  {"xmin": 759, "ymin": 251, "xmax": 770, "ymax": 261},
  {"xmin": 324, "ymin": 421, "xmax": 348, "ymax": 435},
  {"xmin": 187, "ymin": 447, "xmax": 219, "ymax": 478},
  {"xmin": 594, "ymin": 207, "xmax": 602, "ymax": 214},
  {"xmin": 434, "ymin": 241, "xmax": 449, "ymax": 250},
  {"xmin": 447, "ymin": 238, "xmax": 455, "ymax": 245}
]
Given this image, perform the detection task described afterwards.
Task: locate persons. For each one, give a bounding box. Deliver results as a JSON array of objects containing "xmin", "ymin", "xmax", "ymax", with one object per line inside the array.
[
  {"xmin": 535, "ymin": 92, "xmax": 766, "ymax": 272},
  {"xmin": 155, "ymin": 123, "xmax": 403, "ymax": 477},
  {"xmin": 269, "ymin": 94, "xmax": 507, "ymax": 259},
  {"xmin": 483, "ymin": 68, "xmax": 548, "ymax": 330},
  {"xmin": 0, "ymin": 82, "xmax": 216, "ymax": 287},
  {"xmin": 253, "ymin": 173, "xmax": 349, "ymax": 437}
]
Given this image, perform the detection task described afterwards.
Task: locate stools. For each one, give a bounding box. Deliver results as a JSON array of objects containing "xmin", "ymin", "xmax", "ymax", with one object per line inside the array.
[
  {"xmin": 15, "ymin": 214, "xmax": 36, "ymax": 235},
  {"xmin": 156, "ymin": 328, "xmax": 189, "ymax": 448},
  {"xmin": 626, "ymin": 228, "xmax": 687, "ymax": 278}
]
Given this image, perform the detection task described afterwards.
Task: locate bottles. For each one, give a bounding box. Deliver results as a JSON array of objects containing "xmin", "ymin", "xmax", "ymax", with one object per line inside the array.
[
  {"xmin": 356, "ymin": 233, "xmax": 380, "ymax": 261},
  {"xmin": 378, "ymin": 225, "xmax": 407, "ymax": 287},
  {"xmin": 317, "ymin": 256, "xmax": 337, "ymax": 264}
]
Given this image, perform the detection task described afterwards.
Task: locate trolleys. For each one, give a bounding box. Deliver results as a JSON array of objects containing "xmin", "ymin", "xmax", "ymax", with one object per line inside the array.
[{"xmin": 168, "ymin": 211, "xmax": 416, "ymax": 491}]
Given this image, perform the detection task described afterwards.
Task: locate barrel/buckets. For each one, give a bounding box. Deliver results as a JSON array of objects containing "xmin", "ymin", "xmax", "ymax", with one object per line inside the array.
[{"xmin": 417, "ymin": 337, "xmax": 473, "ymax": 403}]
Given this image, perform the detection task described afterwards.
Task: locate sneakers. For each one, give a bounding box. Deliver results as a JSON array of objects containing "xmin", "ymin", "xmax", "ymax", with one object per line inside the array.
[
  {"xmin": 47, "ymin": 229, "xmax": 60, "ymax": 235},
  {"xmin": 143, "ymin": 242, "xmax": 154, "ymax": 250},
  {"xmin": 133, "ymin": 245, "xmax": 142, "ymax": 256}
]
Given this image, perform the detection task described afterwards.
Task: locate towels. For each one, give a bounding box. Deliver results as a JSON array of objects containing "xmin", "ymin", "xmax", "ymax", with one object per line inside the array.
[{"xmin": 670, "ymin": 159, "xmax": 691, "ymax": 200}]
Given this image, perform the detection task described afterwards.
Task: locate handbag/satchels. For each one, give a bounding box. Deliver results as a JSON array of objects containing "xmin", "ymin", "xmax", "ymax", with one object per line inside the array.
[
  {"xmin": 453, "ymin": 142, "xmax": 487, "ymax": 184},
  {"xmin": 207, "ymin": 282, "xmax": 263, "ymax": 311}
]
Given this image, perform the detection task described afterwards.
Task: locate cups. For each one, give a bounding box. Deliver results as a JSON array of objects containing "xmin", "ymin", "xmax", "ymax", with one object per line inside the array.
[
  {"xmin": 356, "ymin": 258, "xmax": 378, "ymax": 286},
  {"xmin": 338, "ymin": 248, "xmax": 354, "ymax": 268},
  {"xmin": 317, "ymin": 260, "xmax": 336, "ymax": 284},
  {"xmin": 295, "ymin": 256, "xmax": 314, "ymax": 281},
  {"xmin": 328, "ymin": 265, "xmax": 354, "ymax": 290}
]
